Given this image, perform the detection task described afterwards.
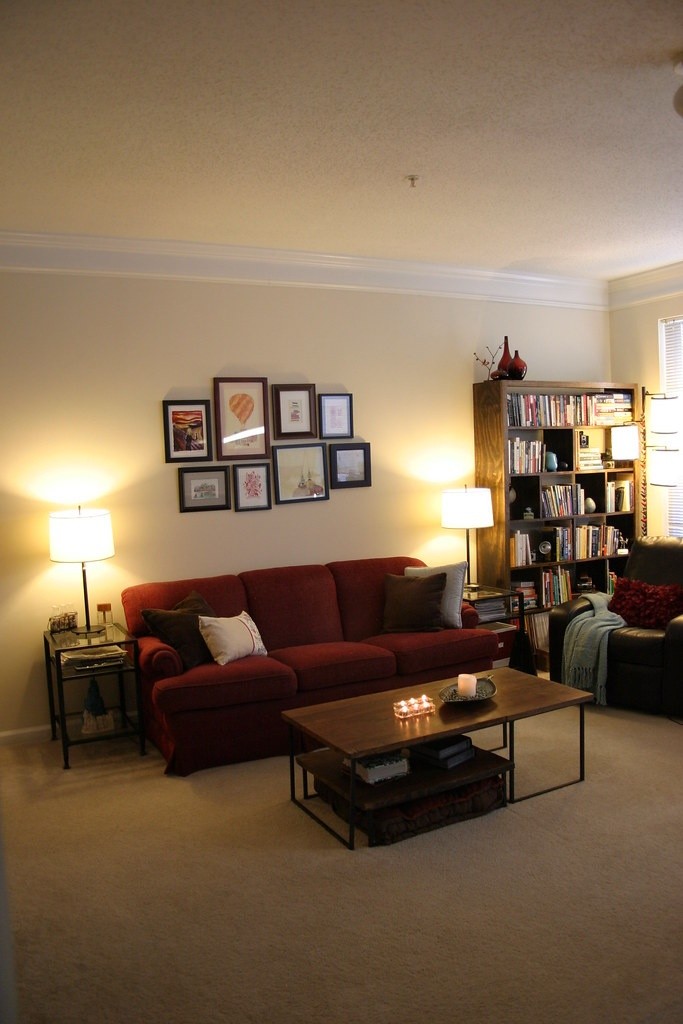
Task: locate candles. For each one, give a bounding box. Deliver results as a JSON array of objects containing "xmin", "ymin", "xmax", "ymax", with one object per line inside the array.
[
  {"xmin": 395, "ymin": 706, "xmax": 411, "ymax": 718},
  {"xmin": 457, "ymin": 674, "xmax": 477, "ymax": 698},
  {"xmin": 417, "ymin": 695, "xmax": 434, "ymax": 705},
  {"xmin": 422, "ymin": 701, "xmax": 435, "ymax": 714},
  {"xmin": 405, "ymin": 697, "xmax": 419, "ymax": 708},
  {"xmin": 393, "ymin": 700, "xmax": 410, "ymax": 713},
  {"xmin": 410, "ymin": 704, "xmax": 423, "ymax": 716}
]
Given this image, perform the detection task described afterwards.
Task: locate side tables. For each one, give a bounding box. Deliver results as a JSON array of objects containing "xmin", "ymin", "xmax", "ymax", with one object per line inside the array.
[
  {"xmin": 43, "ymin": 622, "xmax": 147, "ymax": 771},
  {"xmin": 462, "ymin": 583, "xmax": 526, "ymax": 671}
]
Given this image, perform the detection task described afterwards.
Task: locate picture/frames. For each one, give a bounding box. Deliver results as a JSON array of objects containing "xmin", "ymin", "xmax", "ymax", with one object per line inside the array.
[
  {"xmin": 271, "ymin": 383, "xmax": 318, "ymax": 440},
  {"xmin": 177, "ymin": 465, "xmax": 232, "ymax": 513},
  {"xmin": 161, "ymin": 400, "xmax": 213, "ymax": 463},
  {"xmin": 318, "ymin": 393, "xmax": 354, "ymax": 439},
  {"xmin": 328, "ymin": 441, "xmax": 371, "ymax": 490},
  {"xmin": 232, "ymin": 463, "xmax": 272, "ymax": 512},
  {"xmin": 213, "ymin": 377, "xmax": 271, "ymax": 461},
  {"xmin": 271, "ymin": 443, "xmax": 329, "ymax": 505}
]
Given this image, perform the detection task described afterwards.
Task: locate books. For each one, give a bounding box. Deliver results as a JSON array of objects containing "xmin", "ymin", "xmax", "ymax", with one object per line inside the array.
[
  {"xmin": 542, "ymin": 483, "xmax": 584, "ymax": 518},
  {"xmin": 512, "ymin": 581, "xmax": 538, "ymax": 612},
  {"xmin": 414, "ymin": 734, "xmax": 475, "ymax": 769},
  {"xmin": 341, "ymin": 756, "xmax": 409, "ymax": 783},
  {"xmin": 543, "ymin": 565, "xmax": 592, "ymax": 608},
  {"xmin": 506, "ymin": 392, "xmax": 633, "ymax": 427},
  {"xmin": 508, "ymin": 436, "xmax": 546, "ymax": 474},
  {"xmin": 606, "ymin": 480, "xmax": 633, "ymax": 513},
  {"xmin": 532, "ymin": 526, "xmax": 573, "ymax": 561},
  {"xmin": 510, "ymin": 530, "xmax": 532, "ymax": 567},
  {"xmin": 470, "ymin": 592, "xmax": 507, "ymax": 620},
  {"xmin": 575, "ymin": 522, "xmax": 619, "ymax": 559},
  {"xmin": 576, "ymin": 431, "xmax": 603, "ymax": 470},
  {"xmin": 609, "ymin": 572, "xmax": 617, "ymax": 595}
]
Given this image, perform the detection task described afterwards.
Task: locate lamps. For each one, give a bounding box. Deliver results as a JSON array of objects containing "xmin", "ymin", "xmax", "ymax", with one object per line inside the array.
[
  {"xmin": 49, "ymin": 505, "xmax": 115, "ymax": 638},
  {"xmin": 439, "ymin": 484, "xmax": 495, "ymax": 592}
]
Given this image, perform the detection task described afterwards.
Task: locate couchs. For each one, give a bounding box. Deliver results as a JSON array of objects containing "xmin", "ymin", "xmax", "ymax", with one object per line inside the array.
[
  {"xmin": 548, "ymin": 535, "xmax": 683, "ymax": 726},
  {"xmin": 120, "ymin": 556, "xmax": 501, "ymax": 777}
]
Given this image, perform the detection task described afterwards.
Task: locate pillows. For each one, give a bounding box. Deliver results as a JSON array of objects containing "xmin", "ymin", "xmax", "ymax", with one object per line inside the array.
[
  {"xmin": 200, "ymin": 610, "xmax": 268, "ymax": 667},
  {"xmin": 405, "ymin": 561, "xmax": 468, "ymax": 629},
  {"xmin": 138, "ymin": 590, "xmax": 221, "ymax": 670},
  {"xmin": 381, "ymin": 572, "xmax": 447, "ymax": 634},
  {"xmin": 607, "ymin": 578, "xmax": 683, "ymax": 630}
]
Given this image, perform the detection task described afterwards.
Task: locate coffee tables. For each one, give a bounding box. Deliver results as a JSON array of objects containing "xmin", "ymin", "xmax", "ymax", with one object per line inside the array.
[{"xmin": 282, "ymin": 668, "xmax": 595, "ymax": 851}]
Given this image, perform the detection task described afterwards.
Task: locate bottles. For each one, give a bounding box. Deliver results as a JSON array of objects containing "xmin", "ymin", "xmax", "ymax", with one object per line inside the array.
[
  {"xmin": 97, "ymin": 604, "xmax": 113, "ymax": 627},
  {"xmin": 507, "ymin": 350, "xmax": 528, "ymax": 380},
  {"xmin": 498, "ymin": 336, "xmax": 513, "ymax": 376}
]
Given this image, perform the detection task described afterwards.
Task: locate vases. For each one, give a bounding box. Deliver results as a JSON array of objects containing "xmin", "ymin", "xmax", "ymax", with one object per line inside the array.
[
  {"xmin": 507, "ymin": 350, "xmax": 526, "ymax": 380},
  {"xmin": 497, "ymin": 336, "xmax": 512, "ymax": 376},
  {"xmin": 490, "ymin": 365, "xmax": 509, "ymax": 380},
  {"xmin": 584, "ymin": 498, "xmax": 597, "ymax": 514}
]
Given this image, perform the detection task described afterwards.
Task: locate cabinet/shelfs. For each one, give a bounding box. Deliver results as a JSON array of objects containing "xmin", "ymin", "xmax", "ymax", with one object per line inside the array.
[{"xmin": 472, "ymin": 380, "xmax": 643, "ymax": 658}]
